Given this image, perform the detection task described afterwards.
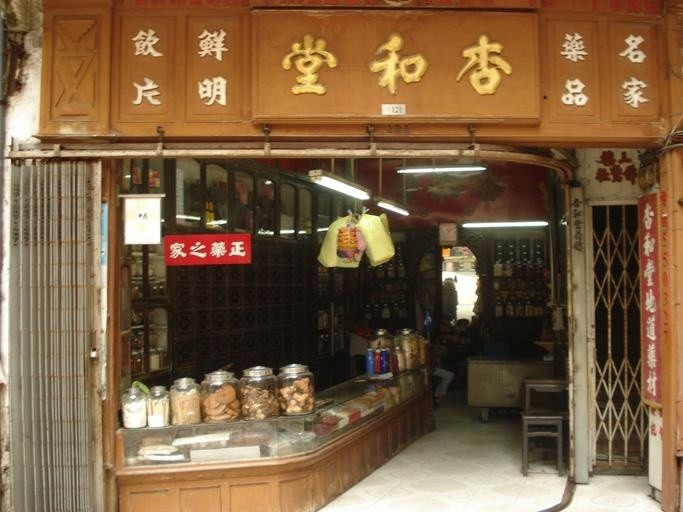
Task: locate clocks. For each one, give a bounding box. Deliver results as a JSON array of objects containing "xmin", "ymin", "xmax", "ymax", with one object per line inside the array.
[{"xmin": 438, "ymin": 222, "xmax": 459, "ymax": 246}]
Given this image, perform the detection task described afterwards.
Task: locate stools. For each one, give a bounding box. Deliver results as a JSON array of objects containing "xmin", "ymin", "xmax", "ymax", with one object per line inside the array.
[
  {"xmin": 522, "ymin": 377, "xmax": 568, "ymax": 420},
  {"xmin": 519, "ymin": 410, "xmax": 566, "ymax": 476}
]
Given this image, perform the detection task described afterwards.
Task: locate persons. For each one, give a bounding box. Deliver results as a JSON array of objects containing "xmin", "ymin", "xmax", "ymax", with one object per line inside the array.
[{"xmin": 432, "ymin": 366, "xmax": 454, "ymax": 404}]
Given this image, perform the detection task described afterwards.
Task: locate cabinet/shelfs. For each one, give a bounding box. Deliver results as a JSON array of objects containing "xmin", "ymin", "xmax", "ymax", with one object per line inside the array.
[
  {"xmin": 117, "ymin": 235, "xmax": 435, "ymax": 510},
  {"xmin": 467, "ymin": 360, "xmax": 555, "ymax": 423},
  {"xmin": 484, "ymin": 233, "xmax": 549, "ymax": 328}
]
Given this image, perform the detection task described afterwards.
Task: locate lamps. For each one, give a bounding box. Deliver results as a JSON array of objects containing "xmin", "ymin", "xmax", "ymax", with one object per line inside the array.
[
  {"xmin": 306, "ymin": 158, "xmax": 370, "ymax": 203},
  {"xmin": 375, "ymin": 159, "xmax": 409, "ymax": 218},
  {"xmin": 394, "ymin": 158, "xmax": 486, "ymax": 176}
]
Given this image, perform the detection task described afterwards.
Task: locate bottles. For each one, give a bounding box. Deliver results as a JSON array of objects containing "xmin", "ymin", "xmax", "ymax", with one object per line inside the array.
[
  {"xmin": 366, "ymin": 246, "xmax": 428, "ymax": 377},
  {"xmin": 318, "ymin": 263, "xmax": 343, "ymax": 354},
  {"xmin": 493, "ymin": 259, "xmax": 550, "ymax": 318},
  {"xmin": 121, "ymin": 363, "xmax": 316, "ymax": 429},
  {"xmin": 131, "ymin": 259, "xmax": 167, "ymax": 372},
  {"xmin": 124, "ymin": 164, "xmax": 274, "ymax": 237}
]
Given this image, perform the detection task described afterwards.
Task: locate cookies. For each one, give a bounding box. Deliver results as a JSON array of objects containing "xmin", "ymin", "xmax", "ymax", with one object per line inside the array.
[{"xmin": 203, "ymin": 385, "xmax": 240, "ymax": 421}]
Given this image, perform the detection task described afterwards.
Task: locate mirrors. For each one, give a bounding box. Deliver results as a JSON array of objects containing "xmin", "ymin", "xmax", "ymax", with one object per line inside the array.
[
  {"xmin": 436, "ymin": 242, "xmax": 483, "ymax": 326},
  {"xmin": 124, "ymin": 158, "xmax": 345, "ymax": 240}
]
